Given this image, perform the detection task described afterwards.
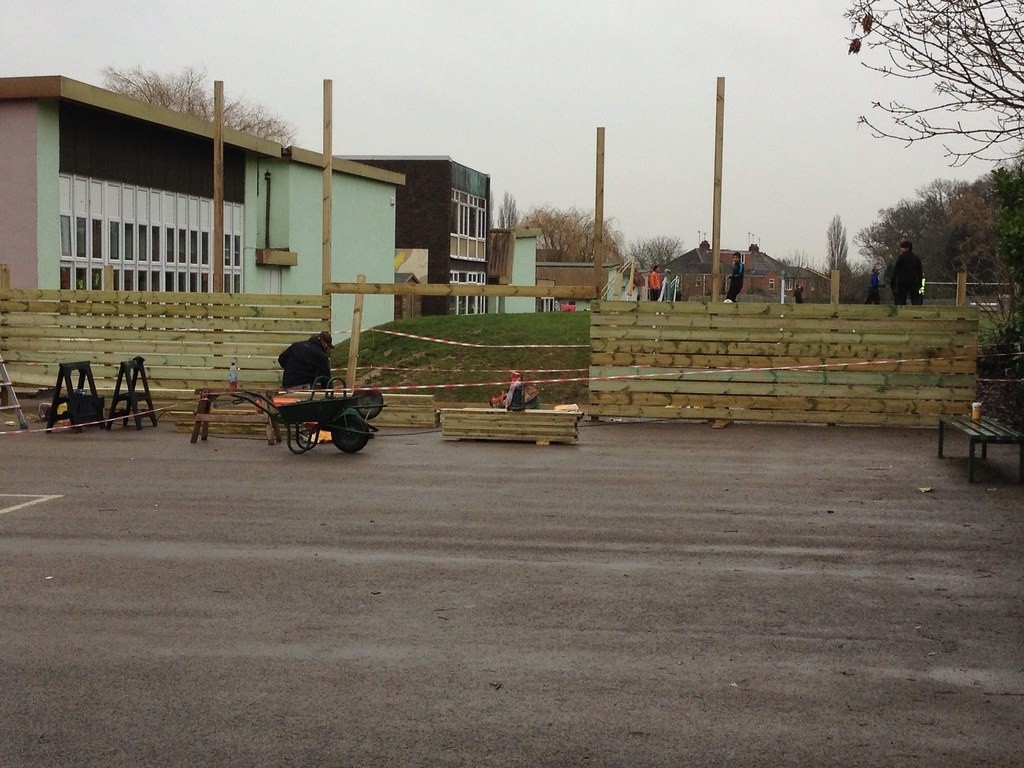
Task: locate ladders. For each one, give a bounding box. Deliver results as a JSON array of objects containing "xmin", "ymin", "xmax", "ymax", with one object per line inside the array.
[{"xmin": 0, "ymin": 352, "xmax": 29, "ymax": 429}]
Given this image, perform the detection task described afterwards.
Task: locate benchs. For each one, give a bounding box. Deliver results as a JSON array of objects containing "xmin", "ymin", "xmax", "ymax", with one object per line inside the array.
[
  {"xmin": 436, "ymin": 406, "xmax": 585, "ymax": 445},
  {"xmin": 191, "ymin": 387, "xmax": 281, "ymax": 445},
  {"xmin": 938, "ymin": 414, "xmax": 1024, "ymax": 484},
  {"xmin": 174, "ymin": 395, "xmax": 436, "ymax": 436}
]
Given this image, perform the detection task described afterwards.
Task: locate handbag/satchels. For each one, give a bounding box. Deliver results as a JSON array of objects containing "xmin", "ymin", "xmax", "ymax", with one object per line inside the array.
[{"xmin": 67, "ymin": 391, "xmax": 97, "ymax": 423}]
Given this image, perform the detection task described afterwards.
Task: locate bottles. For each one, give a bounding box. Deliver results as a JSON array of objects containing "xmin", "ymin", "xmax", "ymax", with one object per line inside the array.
[{"xmin": 228, "ymin": 362, "xmax": 237, "ymax": 391}]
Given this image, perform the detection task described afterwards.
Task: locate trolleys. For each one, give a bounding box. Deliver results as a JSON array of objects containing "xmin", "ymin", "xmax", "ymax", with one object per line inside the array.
[{"xmin": 201, "ymin": 375, "xmax": 389, "ymax": 454}]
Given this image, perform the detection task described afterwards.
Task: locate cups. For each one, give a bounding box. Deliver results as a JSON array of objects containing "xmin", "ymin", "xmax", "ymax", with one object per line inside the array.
[{"xmin": 972, "ymin": 403, "xmax": 982, "ymax": 420}]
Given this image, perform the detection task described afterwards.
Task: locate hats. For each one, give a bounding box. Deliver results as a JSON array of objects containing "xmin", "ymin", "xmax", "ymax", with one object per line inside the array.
[
  {"xmin": 872, "ymin": 268, "xmax": 879, "ymax": 273},
  {"xmin": 732, "ymin": 252, "xmax": 741, "ymax": 258},
  {"xmin": 320, "ymin": 332, "xmax": 334, "ymax": 349}
]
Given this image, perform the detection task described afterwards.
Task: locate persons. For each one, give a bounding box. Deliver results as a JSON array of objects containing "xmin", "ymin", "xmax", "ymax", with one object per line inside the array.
[
  {"xmin": 890, "ymin": 240, "xmax": 924, "ymax": 305},
  {"xmin": 277, "ymin": 330, "xmax": 335, "ymax": 390},
  {"xmin": 726, "ymin": 251, "xmax": 744, "ymax": 303},
  {"xmin": 672, "ymin": 287, "xmax": 682, "ymax": 301},
  {"xmin": 793, "ymin": 285, "xmax": 804, "ymax": 304},
  {"xmin": 649, "ymin": 265, "xmax": 661, "ymax": 301},
  {"xmin": 864, "ymin": 268, "xmax": 887, "ymax": 304}
]
